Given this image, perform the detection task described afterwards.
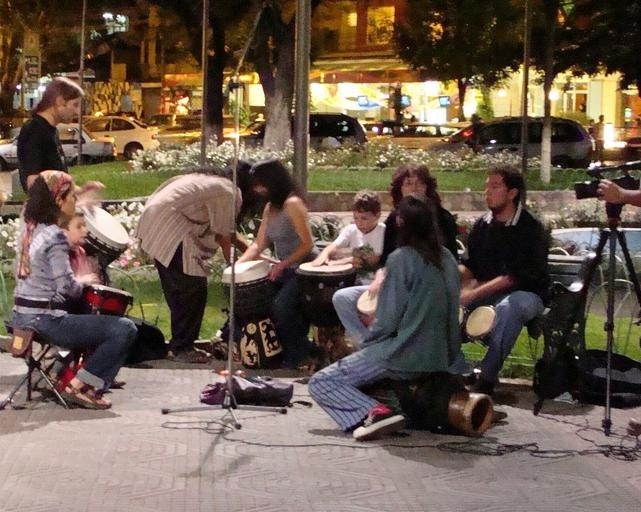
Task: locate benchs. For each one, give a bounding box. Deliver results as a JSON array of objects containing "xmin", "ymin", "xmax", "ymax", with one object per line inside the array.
[{"xmin": 456, "ymin": 249, "xmax": 600, "ymax": 357}]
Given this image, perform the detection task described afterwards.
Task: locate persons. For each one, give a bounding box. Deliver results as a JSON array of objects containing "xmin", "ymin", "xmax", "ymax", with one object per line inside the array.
[
  {"xmin": 60, "ymin": 212, "xmax": 126, "ymax": 388},
  {"xmin": 17, "ymin": 77, "xmax": 107, "ymax": 195},
  {"xmin": 308, "ymin": 196, "xmax": 465, "ymax": 441},
  {"xmin": 597, "ymin": 178, "xmax": 641, "ymax": 435},
  {"xmin": 457, "ymin": 164, "xmax": 550, "ymax": 393},
  {"xmin": 593, "ymin": 116, "xmax": 606, "ymax": 166},
  {"xmin": 331, "ymin": 165, "xmax": 457, "ymax": 351},
  {"xmin": 235, "ymin": 158, "xmax": 320, "ymax": 367},
  {"xmin": 135, "ymin": 158, "xmax": 281, "ymax": 363},
  {"xmin": 312, "ymin": 190, "xmax": 387, "ymax": 285},
  {"xmin": 9, "ymin": 169, "xmax": 136, "ymax": 410}
]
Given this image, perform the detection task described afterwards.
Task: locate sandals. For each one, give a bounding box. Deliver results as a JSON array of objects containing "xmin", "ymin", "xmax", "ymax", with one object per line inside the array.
[{"xmin": 61, "ymin": 382, "xmax": 112, "ymax": 409}]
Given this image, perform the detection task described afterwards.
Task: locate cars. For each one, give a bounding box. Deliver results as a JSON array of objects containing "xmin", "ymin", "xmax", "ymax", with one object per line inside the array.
[
  {"xmin": 618, "ymin": 138, "xmax": 641, "ymax": 159},
  {"xmin": 0, "ymin": 124, "xmax": 118, "ymax": 168},
  {"xmin": 85, "ymin": 115, "xmax": 161, "ymax": 162}
]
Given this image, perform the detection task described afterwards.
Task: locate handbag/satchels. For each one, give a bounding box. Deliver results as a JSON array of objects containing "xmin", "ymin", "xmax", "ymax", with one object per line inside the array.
[
  {"xmin": 231, "ymin": 375, "xmax": 293, "ymax": 406},
  {"xmin": 200, "ymin": 383, "xmax": 226, "ymax": 405}
]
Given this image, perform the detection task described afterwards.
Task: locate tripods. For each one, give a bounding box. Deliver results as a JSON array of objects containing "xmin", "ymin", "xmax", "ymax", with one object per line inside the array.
[
  {"xmin": 161, "ymin": 0, "xmax": 288, "ymax": 430},
  {"xmin": 531, "ymin": 223, "xmax": 641, "ymax": 436}
]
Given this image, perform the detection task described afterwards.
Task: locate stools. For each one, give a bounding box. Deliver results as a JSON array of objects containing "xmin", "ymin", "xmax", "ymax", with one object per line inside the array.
[{"xmin": 0, "ymin": 321, "xmax": 79, "ymax": 412}]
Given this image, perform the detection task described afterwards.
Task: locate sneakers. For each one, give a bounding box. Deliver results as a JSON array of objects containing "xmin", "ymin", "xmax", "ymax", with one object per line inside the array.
[
  {"xmin": 353, "ymin": 409, "xmax": 405, "ymax": 440},
  {"xmin": 168, "ymin": 346, "xmax": 208, "ymax": 364},
  {"xmin": 463, "ymin": 373, "xmax": 495, "ymax": 394}
]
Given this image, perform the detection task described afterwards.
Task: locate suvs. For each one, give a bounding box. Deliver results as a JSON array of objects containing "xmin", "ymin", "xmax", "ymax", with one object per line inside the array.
[
  {"xmin": 237, "ymin": 112, "xmax": 369, "ymax": 149},
  {"xmin": 419, "ymin": 115, "xmax": 593, "ymax": 170}
]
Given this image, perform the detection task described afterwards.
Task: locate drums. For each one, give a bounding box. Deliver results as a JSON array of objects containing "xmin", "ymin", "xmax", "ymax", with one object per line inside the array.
[
  {"xmin": 68, "ymin": 200, "xmax": 128, "ymax": 269},
  {"xmin": 295, "ymin": 261, "xmax": 356, "ymax": 377},
  {"xmin": 356, "ymin": 290, "xmax": 378, "ymax": 327},
  {"xmin": 458, "ymin": 304, "xmax": 498, "ymax": 344},
  {"xmin": 221, "ymin": 260, "xmax": 284, "ymax": 369},
  {"xmin": 55, "ymin": 283, "xmax": 133, "ymax": 395}
]
